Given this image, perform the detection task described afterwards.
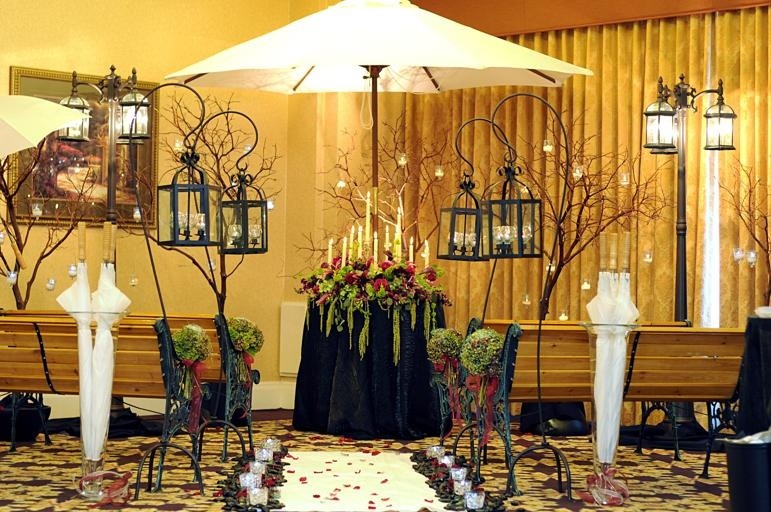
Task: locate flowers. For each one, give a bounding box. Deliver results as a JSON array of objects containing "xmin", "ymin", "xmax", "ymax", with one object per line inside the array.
[{"xmin": 293, "ymin": 258, "xmax": 453, "ymax": 366}]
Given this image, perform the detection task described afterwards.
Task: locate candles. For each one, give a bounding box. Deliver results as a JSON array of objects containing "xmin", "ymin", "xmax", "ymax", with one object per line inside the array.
[
  {"xmin": 385, "ymin": 225, "xmax": 390, "ymax": 260},
  {"xmin": 326, "ymin": 237, "xmax": 334, "ymax": 268},
  {"xmin": 341, "ymin": 236, "xmax": 347, "ymax": 268},
  {"xmin": 357, "ymin": 225, "xmax": 362, "ymax": 259},
  {"xmin": 424, "ymin": 239, "xmax": 431, "ymax": 268},
  {"xmin": 408, "ymin": 236, "xmax": 415, "ymax": 263},
  {"xmin": 348, "ymin": 225, "xmax": 354, "ymax": 257},
  {"xmin": 396, "ymin": 205, "xmax": 403, "ymax": 260},
  {"xmin": 366, "ymin": 190, "xmax": 370, "ymax": 245},
  {"xmin": 373, "ymin": 231, "xmax": 379, "ymax": 266}
]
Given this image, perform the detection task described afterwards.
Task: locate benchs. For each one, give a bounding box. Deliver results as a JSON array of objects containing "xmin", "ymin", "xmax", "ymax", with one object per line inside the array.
[
  {"xmin": 0, "ymin": 317, "xmax": 204, "ymax": 502},
  {"xmin": 428, "ymin": 317, "xmax": 691, "ymax": 465},
  {"xmin": 473, "ymin": 325, "xmax": 746, "ymax": 500},
  {"xmin": 0, "ymin": 309, "xmax": 259, "ymax": 462}
]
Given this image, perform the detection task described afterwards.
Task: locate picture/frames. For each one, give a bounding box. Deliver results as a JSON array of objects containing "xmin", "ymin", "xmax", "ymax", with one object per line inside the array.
[{"xmin": 6, "ymin": 64, "xmax": 162, "ymax": 232}]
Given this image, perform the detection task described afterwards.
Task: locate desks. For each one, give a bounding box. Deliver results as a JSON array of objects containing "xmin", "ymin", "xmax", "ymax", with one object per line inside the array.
[{"xmin": 291, "ymin": 298, "xmax": 454, "ymax": 442}]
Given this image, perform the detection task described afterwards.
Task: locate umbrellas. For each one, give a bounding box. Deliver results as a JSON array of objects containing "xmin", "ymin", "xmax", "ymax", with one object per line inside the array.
[
  {"xmin": 0, "ymin": 95, "xmax": 96, "ymax": 159},
  {"xmin": 91, "ymin": 220, "xmax": 133, "ymax": 468},
  {"xmin": 111, "ymin": 225, "xmax": 118, "ymax": 286},
  {"xmin": 164, "ymin": 1, "xmax": 596, "ymax": 261},
  {"xmin": 58, "ymin": 221, "xmax": 92, "ymax": 489},
  {"xmin": 586, "ymin": 230, "xmax": 641, "ymax": 474}
]
